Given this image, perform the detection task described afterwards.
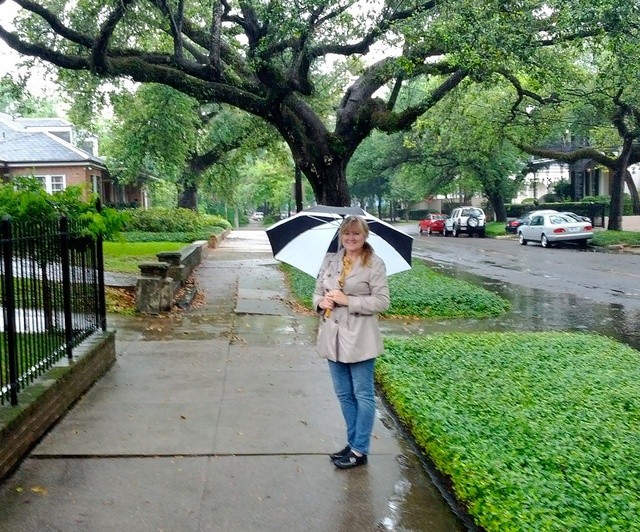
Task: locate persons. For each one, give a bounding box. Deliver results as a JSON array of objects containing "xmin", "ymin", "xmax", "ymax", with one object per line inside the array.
[{"xmin": 312, "ymin": 216, "xmax": 390, "ymax": 468}]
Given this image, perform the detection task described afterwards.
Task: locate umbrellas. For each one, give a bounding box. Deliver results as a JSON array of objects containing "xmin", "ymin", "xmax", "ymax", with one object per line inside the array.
[{"xmin": 265, "ymin": 204, "xmax": 414, "ymax": 317}]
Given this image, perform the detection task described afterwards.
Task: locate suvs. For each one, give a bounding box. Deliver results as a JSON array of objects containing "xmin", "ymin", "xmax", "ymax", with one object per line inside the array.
[
  {"xmin": 252, "ymin": 211, "xmax": 265, "ymax": 221},
  {"xmin": 442, "ymin": 206, "xmax": 486, "ymax": 237}
]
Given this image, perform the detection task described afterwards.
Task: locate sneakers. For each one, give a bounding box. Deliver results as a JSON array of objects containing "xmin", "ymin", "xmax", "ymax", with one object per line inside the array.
[
  {"xmin": 333, "ymin": 451, "xmax": 367, "ymax": 469},
  {"xmin": 330, "ymin": 445, "xmax": 351, "ymax": 460}
]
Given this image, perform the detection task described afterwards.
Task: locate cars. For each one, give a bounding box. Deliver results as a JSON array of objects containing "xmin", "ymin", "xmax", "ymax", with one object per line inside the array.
[
  {"xmin": 562, "ymin": 211, "xmax": 592, "ymax": 224},
  {"xmin": 506, "ymin": 211, "xmax": 534, "ymax": 233},
  {"xmin": 418, "ymin": 213, "xmax": 449, "ymax": 235},
  {"xmin": 516, "ymin": 209, "xmax": 592, "ymax": 247}
]
